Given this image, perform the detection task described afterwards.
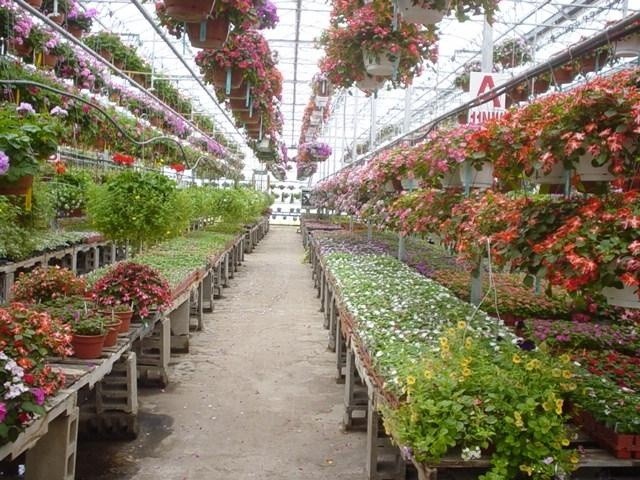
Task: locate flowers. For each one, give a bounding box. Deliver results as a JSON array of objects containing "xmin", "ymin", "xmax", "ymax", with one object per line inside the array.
[
  {"xmin": 329, "ymin": 1, "xmax": 430, "ymax": 49},
  {"xmin": 295, "ymin": 68, "xmax": 640, "ymax": 479}
]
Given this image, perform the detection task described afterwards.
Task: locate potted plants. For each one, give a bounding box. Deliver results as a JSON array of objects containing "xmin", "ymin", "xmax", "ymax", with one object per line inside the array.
[{"xmin": 0, "ymin": 1, "xmax": 283, "ymax": 445}]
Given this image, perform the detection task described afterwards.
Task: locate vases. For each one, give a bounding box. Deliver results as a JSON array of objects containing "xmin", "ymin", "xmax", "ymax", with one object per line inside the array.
[
  {"xmin": 359, "ymin": 39, "xmax": 403, "ymax": 72},
  {"xmin": 398, "ymin": 2, "xmax": 454, "ymax": 25}
]
